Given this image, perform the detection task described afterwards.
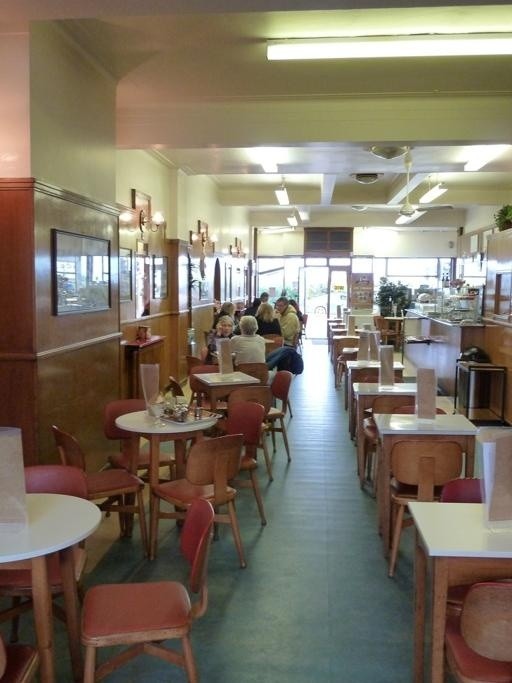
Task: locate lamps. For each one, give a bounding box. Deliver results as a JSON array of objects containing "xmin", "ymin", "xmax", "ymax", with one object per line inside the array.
[
  {"xmin": 368, "ymin": 144, "xmax": 409, "ymax": 160},
  {"xmin": 463, "ymin": 145, "xmax": 511, "ymax": 172},
  {"xmin": 418, "ymin": 181, "xmax": 449, "ymax": 203},
  {"xmin": 201, "ymin": 229, "xmax": 220, "ymax": 249},
  {"xmin": 139, "ymin": 207, "xmax": 165, "ymax": 233},
  {"xmin": 257, "ymin": 162, "xmax": 310, "ymax": 234},
  {"xmin": 265, "ymin": 31, "xmax": 512, "ymax": 65},
  {"xmin": 349, "ymin": 173, "xmax": 384, "ymax": 185},
  {"xmin": 394, "ymin": 209, "xmax": 427, "ymax": 225}
]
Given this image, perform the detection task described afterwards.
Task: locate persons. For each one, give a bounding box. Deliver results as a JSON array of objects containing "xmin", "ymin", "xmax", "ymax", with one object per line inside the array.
[{"xmin": 205, "ymin": 292, "xmax": 304, "ymax": 386}]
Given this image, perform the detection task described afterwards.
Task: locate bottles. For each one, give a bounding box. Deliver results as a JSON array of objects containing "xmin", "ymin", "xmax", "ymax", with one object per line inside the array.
[
  {"xmin": 442, "ymin": 272, "xmax": 450, "ymax": 288},
  {"xmin": 188, "ymin": 337, "xmax": 197, "ymax": 356},
  {"xmin": 194, "ymin": 407, "xmax": 201, "ymax": 420}
]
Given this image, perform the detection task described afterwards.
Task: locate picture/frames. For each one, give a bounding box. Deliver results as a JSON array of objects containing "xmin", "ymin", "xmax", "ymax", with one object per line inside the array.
[
  {"xmin": 131, "ymin": 188, "xmax": 152, "ymax": 214},
  {"xmin": 198, "ymin": 220, "xmax": 208, "ymax": 236},
  {"xmin": 119, "ymin": 247, "xmax": 133, "ymax": 304},
  {"xmin": 190, "ymin": 231, "xmax": 196, "ymax": 244},
  {"xmin": 50, "ymin": 228, "xmax": 113, "ymax": 316},
  {"xmin": 225, "ymin": 261, "xmax": 233, "ymax": 302},
  {"xmin": 153, "ymin": 255, "xmax": 169, "ymax": 299}
]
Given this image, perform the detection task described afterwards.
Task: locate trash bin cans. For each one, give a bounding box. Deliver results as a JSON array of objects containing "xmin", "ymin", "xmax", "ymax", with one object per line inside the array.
[{"xmin": 457, "ymin": 347, "xmax": 491, "ymax": 408}]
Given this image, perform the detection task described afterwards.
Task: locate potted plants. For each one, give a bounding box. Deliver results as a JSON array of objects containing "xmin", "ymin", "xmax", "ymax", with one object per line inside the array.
[{"xmin": 493, "ymin": 202, "xmax": 512, "ymax": 232}]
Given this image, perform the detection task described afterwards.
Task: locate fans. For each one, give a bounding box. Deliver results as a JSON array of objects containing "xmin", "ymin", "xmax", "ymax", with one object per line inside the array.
[{"xmin": 363, "ymin": 147, "xmax": 454, "ymax": 218}]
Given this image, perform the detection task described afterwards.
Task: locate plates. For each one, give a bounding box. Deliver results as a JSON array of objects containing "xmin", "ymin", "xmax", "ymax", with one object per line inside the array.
[{"xmin": 159, "ymin": 407, "xmax": 223, "ymax": 425}]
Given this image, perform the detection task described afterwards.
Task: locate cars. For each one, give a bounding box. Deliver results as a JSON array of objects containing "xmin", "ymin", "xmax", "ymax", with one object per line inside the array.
[
  {"xmin": 372, "ymin": 289, "xmax": 380, "ymax": 314},
  {"xmin": 307, "ymin": 290, "xmax": 347, "ymax": 317}
]
